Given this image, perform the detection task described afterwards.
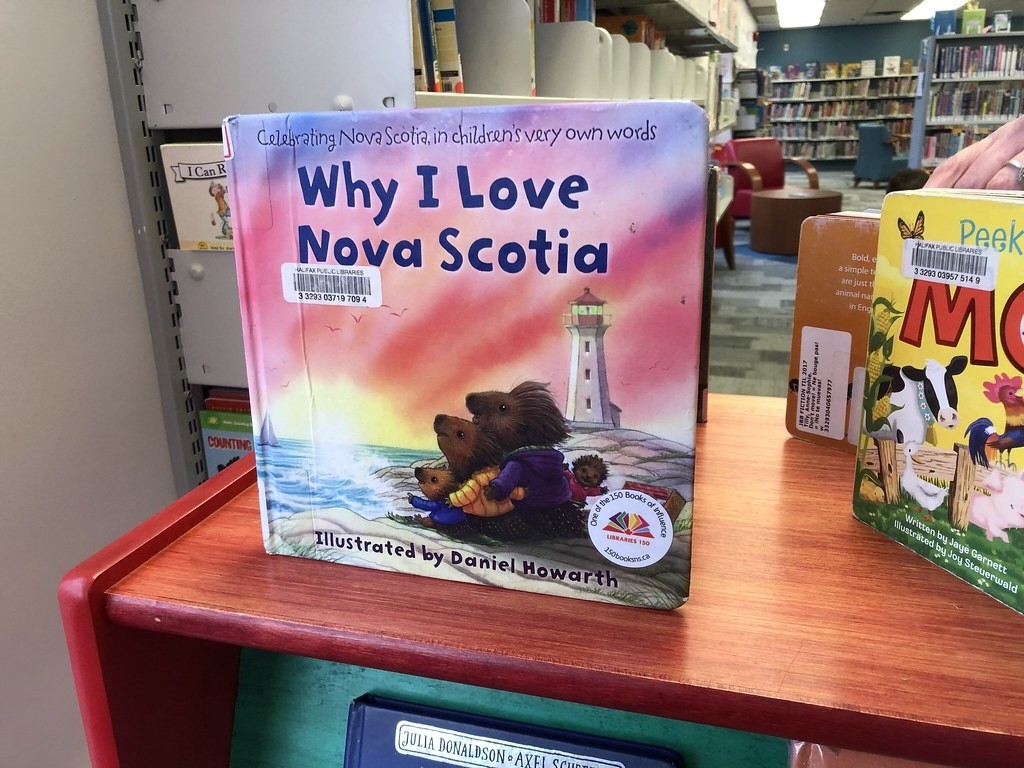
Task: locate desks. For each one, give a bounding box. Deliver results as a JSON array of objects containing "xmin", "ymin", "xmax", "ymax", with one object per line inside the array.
[
  {"xmin": 57, "ymin": 392, "xmax": 1024, "ymax": 768},
  {"xmin": 751, "ymin": 187, "xmax": 843, "ymax": 256}
]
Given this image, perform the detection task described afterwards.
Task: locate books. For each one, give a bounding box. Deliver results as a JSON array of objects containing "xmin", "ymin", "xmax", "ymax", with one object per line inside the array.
[
  {"xmin": 785, "ymin": 188, "xmax": 1024, "ymax": 616},
  {"xmin": 342, "ymin": 692, "xmax": 697, "ymax": 768},
  {"xmin": 790, "ymin": 739, "xmax": 987, "ymax": 768},
  {"xmin": 733, "ymin": 55, "xmax": 921, "ymax": 159},
  {"xmin": 216, "ymin": 99, "xmax": 718, "ymax": 610},
  {"xmin": 408, "ymin": 0, "xmax": 740, "ymax": 116},
  {"xmin": 196, "ymin": 389, "xmax": 254, "ymax": 481},
  {"xmin": 921, "ymin": 0, "xmax": 1024, "ymax": 160}
]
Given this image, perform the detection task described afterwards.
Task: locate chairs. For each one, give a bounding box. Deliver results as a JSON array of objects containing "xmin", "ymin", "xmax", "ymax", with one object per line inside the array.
[
  {"xmin": 852, "ymin": 123, "xmax": 910, "ymax": 190},
  {"xmin": 722, "ymin": 136, "xmax": 821, "ymax": 233}
]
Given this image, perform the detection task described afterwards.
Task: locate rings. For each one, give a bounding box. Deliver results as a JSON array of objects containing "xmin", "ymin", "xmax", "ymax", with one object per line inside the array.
[{"xmin": 1002, "ymin": 157, "xmax": 1024, "ymax": 186}]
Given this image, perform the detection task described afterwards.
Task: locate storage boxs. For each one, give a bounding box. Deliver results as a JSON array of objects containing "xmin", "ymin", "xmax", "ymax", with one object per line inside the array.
[{"xmin": 929, "ymin": 9, "xmax": 985, "ymax": 36}]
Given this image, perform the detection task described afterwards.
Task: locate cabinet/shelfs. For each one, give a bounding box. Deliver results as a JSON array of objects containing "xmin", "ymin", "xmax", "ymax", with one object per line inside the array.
[
  {"xmin": 95, "ymin": 0, "xmax": 743, "ymax": 503},
  {"xmin": 907, "ymin": 30, "xmax": 1024, "ymax": 171},
  {"xmin": 766, "ymin": 71, "xmax": 924, "ymax": 173}
]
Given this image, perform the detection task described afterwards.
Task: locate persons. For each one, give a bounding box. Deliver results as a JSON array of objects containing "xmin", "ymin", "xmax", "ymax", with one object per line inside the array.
[{"xmin": 875, "ymin": 114, "xmax": 1024, "ymax": 204}]
